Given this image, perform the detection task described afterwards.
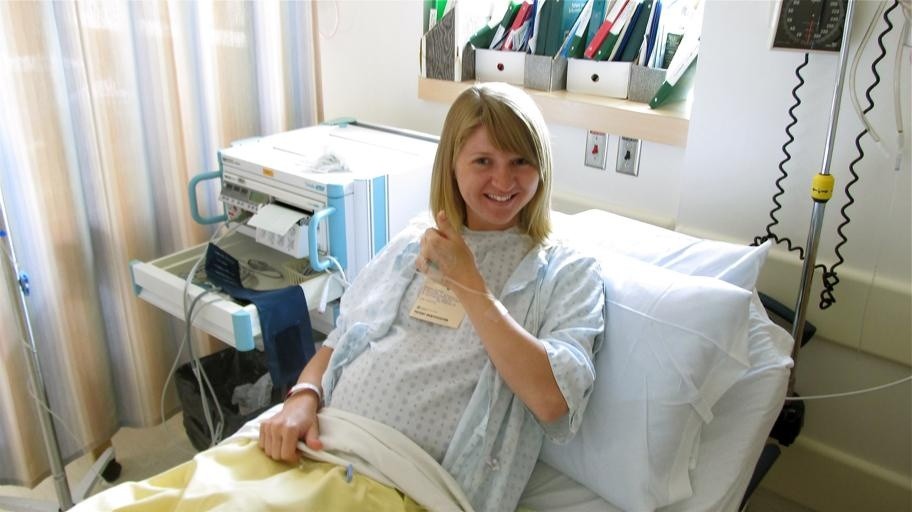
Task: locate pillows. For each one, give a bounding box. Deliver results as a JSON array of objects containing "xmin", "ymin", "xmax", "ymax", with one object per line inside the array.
[
  {"xmin": 454, "ymin": 210, "xmax": 755, "ymax": 510},
  {"xmin": 559, "ymin": 209, "xmax": 772, "ymax": 294}
]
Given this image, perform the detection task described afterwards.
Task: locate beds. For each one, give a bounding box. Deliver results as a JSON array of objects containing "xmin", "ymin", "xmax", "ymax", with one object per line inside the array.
[{"xmin": 62, "ymin": 204, "xmax": 814, "ymax": 512}]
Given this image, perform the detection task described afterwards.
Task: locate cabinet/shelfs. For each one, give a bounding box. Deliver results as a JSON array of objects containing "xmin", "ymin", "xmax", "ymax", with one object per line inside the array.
[{"xmin": 126, "ymin": 117, "xmax": 440, "ymax": 353}]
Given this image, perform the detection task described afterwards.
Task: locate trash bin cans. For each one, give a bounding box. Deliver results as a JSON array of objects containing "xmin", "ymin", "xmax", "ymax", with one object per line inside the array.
[{"xmin": 175, "ymin": 346, "xmax": 283, "ymax": 451}]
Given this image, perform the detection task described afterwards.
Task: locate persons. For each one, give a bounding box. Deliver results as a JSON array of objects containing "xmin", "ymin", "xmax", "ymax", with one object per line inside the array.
[{"xmin": 259, "ymin": 82, "xmax": 604, "ymax": 512}]
